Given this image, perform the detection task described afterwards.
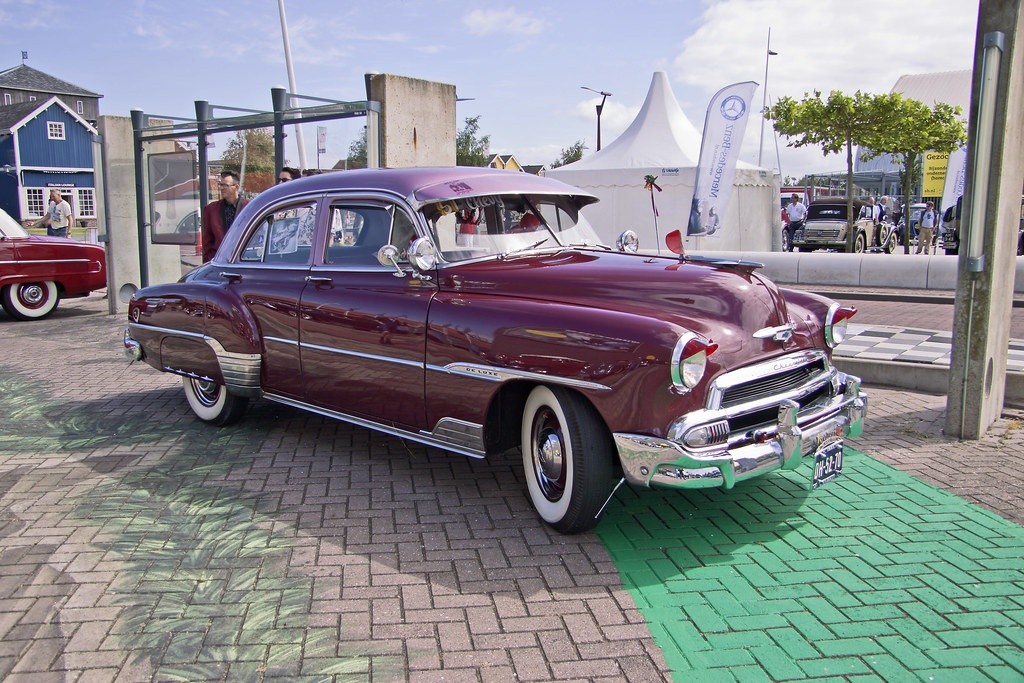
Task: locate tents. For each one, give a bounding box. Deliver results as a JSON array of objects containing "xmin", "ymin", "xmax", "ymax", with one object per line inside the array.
[{"xmin": 538, "ymin": 70, "xmax": 786, "ymax": 254}]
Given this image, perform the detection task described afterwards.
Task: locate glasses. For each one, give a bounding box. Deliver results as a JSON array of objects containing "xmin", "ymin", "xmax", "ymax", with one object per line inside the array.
[
  {"xmin": 276, "ymin": 177, "xmax": 291, "ymax": 183},
  {"xmin": 218, "ymin": 183, "xmax": 235, "ymax": 189}
]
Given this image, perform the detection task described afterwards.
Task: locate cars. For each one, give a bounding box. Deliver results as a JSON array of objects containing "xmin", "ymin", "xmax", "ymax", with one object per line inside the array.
[
  {"xmin": 794, "ymin": 197, "xmax": 899, "ymax": 254},
  {"xmin": 0, "ymin": 208, "xmax": 108, "ymax": 322},
  {"xmin": 118, "ymin": 164, "xmax": 868, "ymax": 535}
]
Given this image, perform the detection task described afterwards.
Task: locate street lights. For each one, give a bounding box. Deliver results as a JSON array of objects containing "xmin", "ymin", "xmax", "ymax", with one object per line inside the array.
[
  {"xmin": 579, "ymin": 85, "xmax": 612, "ymax": 153},
  {"xmin": 756, "ymin": 26, "xmax": 779, "ymax": 166}
]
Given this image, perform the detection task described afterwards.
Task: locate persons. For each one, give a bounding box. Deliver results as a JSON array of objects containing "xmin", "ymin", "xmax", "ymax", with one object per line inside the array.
[
  {"xmin": 262, "ymin": 167, "xmax": 309, "ymax": 252},
  {"xmin": 25, "ymin": 189, "xmax": 73, "ymax": 238},
  {"xmin": 296, "ymin": 168, "xmax": 343, "ymax": 246},
  {"xmin": 870, "ymin": 195, "xmax": 889, "ymax": 253},
  {"xmin": 201, "ymin": 170, "xmax": 250, "ymax": 264},
  {"xmin": 915, "ymin": 201, "xmax": 939, "ymax": 255},
  {"xmin": 857, "ymin": 196, "xmax": 875, "ymax": 219},
  {"xmin": 786, "ymin": 194, "xmax": 809, "ymax": 252}
]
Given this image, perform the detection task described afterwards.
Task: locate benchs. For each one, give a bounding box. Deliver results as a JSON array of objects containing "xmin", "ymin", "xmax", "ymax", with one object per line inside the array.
[{"xmin": 265, "ymin": 246, "xmax": 383, "ymax": 268}]
